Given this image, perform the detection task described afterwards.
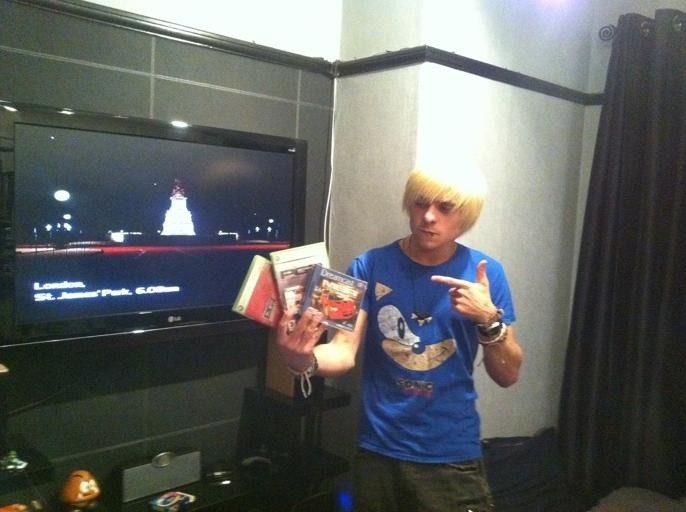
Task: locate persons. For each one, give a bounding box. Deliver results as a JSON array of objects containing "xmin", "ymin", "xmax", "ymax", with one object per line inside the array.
[{"xmin": 275, "ymin": 158, "xmax": 524, "ymax": 507}]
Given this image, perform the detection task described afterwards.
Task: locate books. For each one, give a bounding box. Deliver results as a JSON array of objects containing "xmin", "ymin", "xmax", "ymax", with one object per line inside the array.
[
  {"xmin": 300, "ymin": 265, "xmax": 368, "ymax": 336},
  {"xmin": 267, "ymin": 241, "xmax": 330, "ymax": 338},
  {"xmin": 233, "ymin": 254, "xmax": 282, "ymax": 329}
]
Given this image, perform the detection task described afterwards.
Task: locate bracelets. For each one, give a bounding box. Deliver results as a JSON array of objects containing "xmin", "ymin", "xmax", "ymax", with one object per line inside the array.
[
  {"xmin": 282, "ymin": 354, "xmax": 319, "ymax": 399},
  {"xmin": 477, "ymin": 323, "xmax": 507, "ymax": 346}
]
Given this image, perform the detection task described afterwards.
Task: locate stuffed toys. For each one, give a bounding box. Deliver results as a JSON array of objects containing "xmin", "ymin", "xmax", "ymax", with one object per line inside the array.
[{"xmin": 60, "ymin": 470, "xmax": 100, "ymax": 507}]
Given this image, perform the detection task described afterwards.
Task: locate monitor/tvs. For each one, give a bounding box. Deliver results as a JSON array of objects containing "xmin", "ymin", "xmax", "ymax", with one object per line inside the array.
[{"xmin": 0, "ymin": 96, "xmax": 307, "ymax": 357}]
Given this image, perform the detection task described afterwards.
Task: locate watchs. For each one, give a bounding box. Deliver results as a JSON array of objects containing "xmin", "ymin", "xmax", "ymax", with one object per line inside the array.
[{"xmin": 475, "ymin": 322, "xmax": 500, "ymax": 336}]
